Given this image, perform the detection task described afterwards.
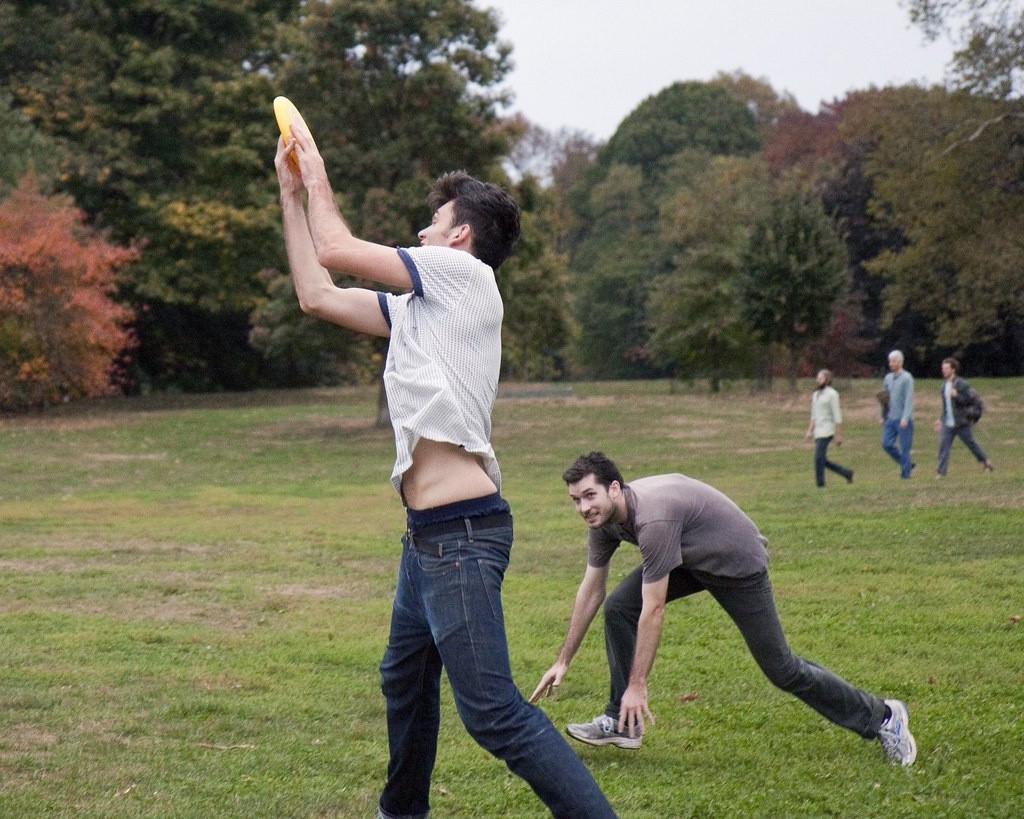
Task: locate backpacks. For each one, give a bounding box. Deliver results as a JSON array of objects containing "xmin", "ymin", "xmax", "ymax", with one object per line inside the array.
[{"xmin": 951, "ymin": 381, "xmax": 983, "ymax": 425}]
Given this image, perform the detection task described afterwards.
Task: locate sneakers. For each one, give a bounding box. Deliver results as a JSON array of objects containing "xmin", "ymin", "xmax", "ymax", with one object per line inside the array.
[
  {"xmin": 567, "ymin": 713, "xmax": 642, "ymax": 748},
  {"xmin": 878, "ymin": 698, "xmax": 916, "ymax": 767}
]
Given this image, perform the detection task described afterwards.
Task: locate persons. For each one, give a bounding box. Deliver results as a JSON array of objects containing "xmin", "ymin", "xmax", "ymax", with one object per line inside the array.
[
  {"xmin": 880, "ymin": 350, "xmax": 916, "ymax": 479},
  {"xmin": 802, "ymin": 369, "xmax": 854, "ymax": 488},
  {"xmin": 933, "ymin": 358, "xmax": 994, "ymax": 480},
  {"xmin": 529, "ymin": 451, "xmax": 918, "ymax": 768},
  {"xmin": 274, "ymin": 124, "xmax": 619, "ymax": 819}
]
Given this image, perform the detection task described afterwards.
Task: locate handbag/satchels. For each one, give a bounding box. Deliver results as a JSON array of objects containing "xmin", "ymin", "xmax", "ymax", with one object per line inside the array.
[{"xmin": 876, "ymin": 391, "xmax": 890, "ymax": 417}]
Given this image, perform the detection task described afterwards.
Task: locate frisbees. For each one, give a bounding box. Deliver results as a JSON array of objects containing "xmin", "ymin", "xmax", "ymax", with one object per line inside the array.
[{"xmin": 271, "ymin": 95, "xmax": 314, "ymax": 160}]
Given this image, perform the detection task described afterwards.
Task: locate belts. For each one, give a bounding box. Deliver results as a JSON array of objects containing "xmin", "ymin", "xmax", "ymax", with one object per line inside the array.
[{"xmin": 406, "ymin": 512, "xmax": 511, "ymax": 557}]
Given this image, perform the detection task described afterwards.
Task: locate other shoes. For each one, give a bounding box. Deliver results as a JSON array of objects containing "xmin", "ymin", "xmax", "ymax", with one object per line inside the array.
[
  {"xmin": 911, "ymin": 463, "xmax": 916, "ymax": 468},
  {"xmin": 935, "ymin": 474, "xmax": 944, "ymax": 480},
  {"xmin": 983, "ymin": 463, "xmax": 993, "ymax": 472},
  {"xmin": 848, "ymin": 468, "xmax": 855, "ymax": 483}
]
